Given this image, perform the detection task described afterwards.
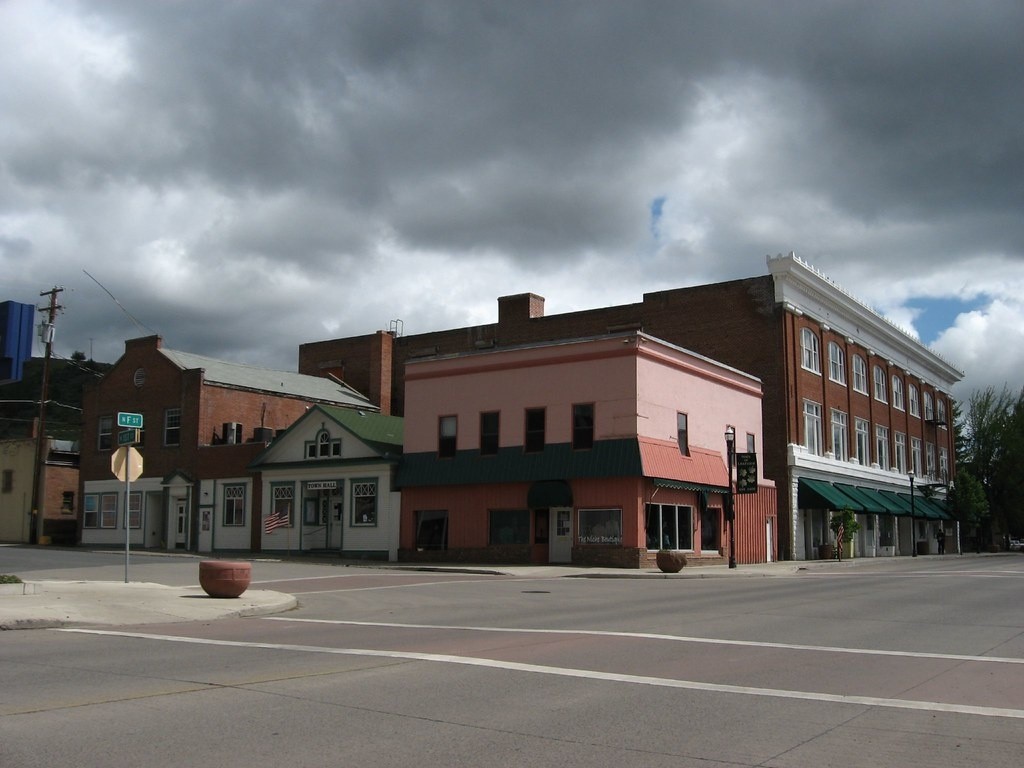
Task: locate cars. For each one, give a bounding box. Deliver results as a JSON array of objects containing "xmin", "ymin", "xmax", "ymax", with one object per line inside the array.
[{"xmin": 1008, "ymin": 537, "xmax": 1024, "ymax": 552}]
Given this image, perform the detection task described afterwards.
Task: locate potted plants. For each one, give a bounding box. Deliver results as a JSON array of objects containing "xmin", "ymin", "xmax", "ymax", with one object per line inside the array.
[{"xmin": 830, "ymin": 507, "xmax": 860, "ymax": 559}]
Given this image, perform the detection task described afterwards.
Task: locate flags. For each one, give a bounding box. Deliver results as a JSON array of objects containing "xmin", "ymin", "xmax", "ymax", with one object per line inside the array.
[
  {"xmin": 263, "ymin": 511, "xmax": 289, "ymax": 534},
  {"xmin": 837, "ymin": 524, "xmax": 844, "ymax": 553}
]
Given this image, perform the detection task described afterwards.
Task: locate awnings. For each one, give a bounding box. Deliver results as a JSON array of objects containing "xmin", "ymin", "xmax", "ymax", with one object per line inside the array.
[{"xmin": 800, "ymin": 477, "xmax": 955, "ymax": 518}]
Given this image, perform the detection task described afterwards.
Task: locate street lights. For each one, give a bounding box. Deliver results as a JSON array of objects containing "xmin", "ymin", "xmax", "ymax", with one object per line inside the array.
[
  {"xmin": 724, "ymin": 425, "xmax": 738, "ymax": 569},
  {"xmin": 908, "ymin": 468, "xmax": 918, "ymax": 558}
]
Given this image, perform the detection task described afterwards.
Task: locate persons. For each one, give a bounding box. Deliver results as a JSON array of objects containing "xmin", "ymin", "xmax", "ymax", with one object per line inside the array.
[{"xmin": 937, "ymin": 528, "xmax": 945, "ymax": 554}]
